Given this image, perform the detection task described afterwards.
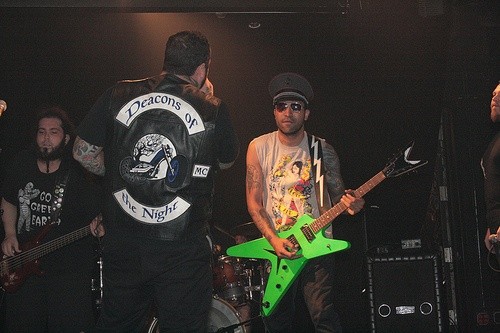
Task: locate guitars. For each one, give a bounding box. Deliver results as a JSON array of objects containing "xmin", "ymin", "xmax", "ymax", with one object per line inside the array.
[
  {"xmin": 0, "ymin": 214, "xmax": 105, "ymax": 293},
  {"xmin": 225, "ymin": 138, "xmax": 428, "ymax": 319}
]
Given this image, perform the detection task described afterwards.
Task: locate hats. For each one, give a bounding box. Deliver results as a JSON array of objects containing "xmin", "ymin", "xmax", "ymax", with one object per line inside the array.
[{"xmin": 267, "ymin": 72, "xmax": 315, "ymax": 108}]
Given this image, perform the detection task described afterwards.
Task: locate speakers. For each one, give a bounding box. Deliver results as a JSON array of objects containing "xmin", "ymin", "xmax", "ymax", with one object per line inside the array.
[{"xmin": 352, "ymin": 254, "xmax": 444, "ymax": 333}]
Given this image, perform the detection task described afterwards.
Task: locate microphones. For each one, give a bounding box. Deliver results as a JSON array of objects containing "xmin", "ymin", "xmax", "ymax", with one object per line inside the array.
[{"xmin": 0, "ymin": 99, "xmax": 7, "ymax": 118}]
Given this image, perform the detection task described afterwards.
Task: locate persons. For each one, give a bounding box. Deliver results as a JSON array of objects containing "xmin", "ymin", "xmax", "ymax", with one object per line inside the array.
[
  {"xmin": 480, "ymin": 83, "xmax": 500, "ymax": 333},
  {"xmin": 245, "ymin": 71, "xmax": 365, "ymax": 333},
  {"xmin": 72, "ymin": 30, "xmax": 241, "ymax": 333},
  {"xmin": 0, "ymin": 106, "xmax": 105, "ymax": 333}
]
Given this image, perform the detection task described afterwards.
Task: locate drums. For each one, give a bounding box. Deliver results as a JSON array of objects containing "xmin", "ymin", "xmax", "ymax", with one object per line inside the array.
[
  {"xmin": 213, "ymin": 258, "xmax": 270, "ymax": 302},
  {"xmin": 148, "ymin": 295, "xmax": 257, "ymax": 333}
]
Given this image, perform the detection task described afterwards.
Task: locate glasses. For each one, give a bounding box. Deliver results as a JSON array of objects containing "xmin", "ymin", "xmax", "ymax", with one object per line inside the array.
[{"xmin": 273, "ymin": 102, "xmax": 306, "ymax": 113}]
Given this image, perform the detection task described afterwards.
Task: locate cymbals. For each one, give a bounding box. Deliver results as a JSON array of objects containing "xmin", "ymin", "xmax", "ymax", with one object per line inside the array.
[{"xmin": 229, "ymin": 221, "xmax": 259, "ymax": 238}]
